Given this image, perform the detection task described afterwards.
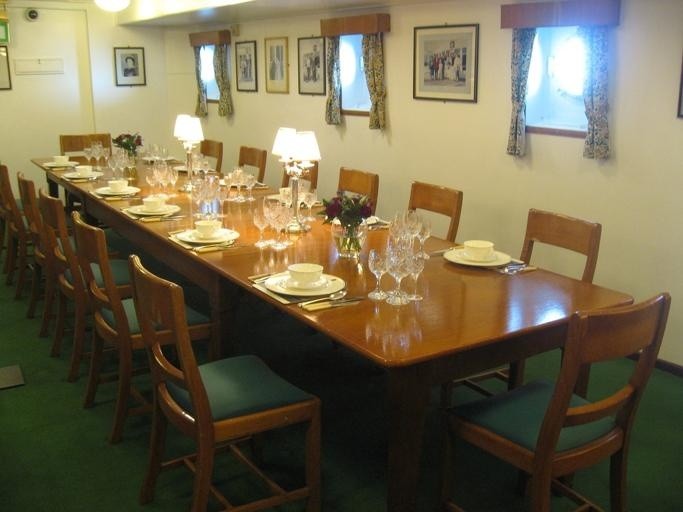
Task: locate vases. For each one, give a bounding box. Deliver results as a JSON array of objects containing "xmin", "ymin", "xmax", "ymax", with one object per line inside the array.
[
  {"xmin": 120, "ymin": 148, "xmax": 143, "ymax": 169},
  {"xmin": 331, "ymin": 217, "xmax": 368, "ymax": 260}
]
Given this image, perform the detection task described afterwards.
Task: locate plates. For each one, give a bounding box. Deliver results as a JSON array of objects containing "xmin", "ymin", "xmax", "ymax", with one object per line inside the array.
[
  {"xmin": 442, "ymin": 249, "xmax": 511, "ymax": 269},
  {"xmin": 264, "ymin": 272, "xmax": 347, "ymax": 296},
  {"xmin": 42, "ymin": 161, "xmax": 103, "ymax": 180}
]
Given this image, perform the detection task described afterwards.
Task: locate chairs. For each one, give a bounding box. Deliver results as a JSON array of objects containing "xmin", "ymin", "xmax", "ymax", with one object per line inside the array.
[
  {"xmin": 439, "ymin": 292, "xmax": 672, "ymax": 511},
  {"xmin": 282, "ymin": 157, "xmax": 318, "ymax": 194},
  {"xmin": 403, "ymin": 180, "xmax": 464, "ymax": 244},
  {"xmin": 336, "ymin": 168, "xmax": 379, "ymax": 217},
  {"xmin": 1, "ymin": 164, "xmax": 131, "ymax": 382},
  {"xmin": 60, "ymin": 134, "xmax": 112, "ymax": 157},
  {"xmin": 126, "ymin": 254, "xmax": 320, "ymax": 511},
  {"xmin": 67, "ymin": 212, "xmax": 215, "ymax": 445},
  {"xmin": 239, "ymin": 146, "xmax": 267, "ymax": 184},
  {"xmin": 447, "ymin": 208, "xmax": 602, "ymax": 402},
  {"xmin": 201, "ymin": 139, "xmax": 223, "ymax": 171}
]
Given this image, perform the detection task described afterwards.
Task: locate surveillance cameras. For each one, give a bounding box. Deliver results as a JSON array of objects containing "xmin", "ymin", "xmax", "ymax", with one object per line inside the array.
[{"xmin": 29, "ymin": 10, "xmax": 37, "ymax": 19}]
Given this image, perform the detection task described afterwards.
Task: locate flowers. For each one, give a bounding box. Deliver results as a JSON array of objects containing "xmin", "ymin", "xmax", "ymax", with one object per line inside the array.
[
  {"xmin": 323, "ymin": 191, "xmax": 375, "ymax": 219},
  {"xmin": 117, "ymin": 134, "xmax": 144, "ymax": 147}
]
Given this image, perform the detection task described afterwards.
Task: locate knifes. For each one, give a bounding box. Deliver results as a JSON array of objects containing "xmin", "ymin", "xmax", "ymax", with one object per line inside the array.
[{"xmin": 302, "ymin": 297, "xmax": 363, "ymax": 311}]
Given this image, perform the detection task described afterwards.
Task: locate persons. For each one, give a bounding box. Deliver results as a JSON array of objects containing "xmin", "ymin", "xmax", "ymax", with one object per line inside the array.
[
  {"xmin": 124, "ymin": 56, "xmax": 138, "ymax": 77},
  {"xmin": 240, "ymin": 55, "xmax": 251, "ymax": 79},
  {"xmin": 429, "ymin": 52, "xmax": 461, "ymax": 81},
  {"xmin": 306, "ymin": 45, "xmax": 320, "ymax": 80},
  {"xmin": 269, "ymin": 56, "xmax": 280, "ymax": 80}
]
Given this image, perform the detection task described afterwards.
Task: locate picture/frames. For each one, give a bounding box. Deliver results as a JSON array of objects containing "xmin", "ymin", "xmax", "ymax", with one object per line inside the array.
[
  {"xmin": 235, "ymin": 36, "xmax": 327, "ymax": 95},
  {"xmin": 0, "ymin": 46, "xmax": 12, "ymax": 91},
  {"xmin": 412, "ymin": 23, "xmax": 480, "ymax": 104},
  {"xmin": 113, "ymin": 46, "xmax": 147, "ymax": 87}
]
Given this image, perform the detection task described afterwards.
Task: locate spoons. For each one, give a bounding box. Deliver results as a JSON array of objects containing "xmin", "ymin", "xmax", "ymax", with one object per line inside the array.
[{"xmin": 297, "ymin": 290, "xmax": 346, "ymax": 306}]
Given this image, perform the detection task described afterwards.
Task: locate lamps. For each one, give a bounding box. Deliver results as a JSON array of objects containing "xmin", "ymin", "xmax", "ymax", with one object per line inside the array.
[
  {"xmin": 174, "ymin": 113, "xmax": 205, "ymax": 193},
  {"xmin": 271, "ymin": 126, "xmax": 322, "ymax": 233}
]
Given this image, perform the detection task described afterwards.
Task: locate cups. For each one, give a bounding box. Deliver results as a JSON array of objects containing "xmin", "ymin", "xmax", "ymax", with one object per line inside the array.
[
  {"xmin": 463, "ymin": 239, "xmax": 495, "ymax": 260},
  {"xmin": 54, "ymin": 155, "xmax": 69, "ymax": 163},
  {"xmin": 288, "ymin": 262, "xmax": 323, "ymax": 284}
]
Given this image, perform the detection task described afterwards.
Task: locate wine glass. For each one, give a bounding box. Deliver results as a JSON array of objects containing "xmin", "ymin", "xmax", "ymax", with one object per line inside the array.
[
  {"xmin": 83, "ymin": 138, "xmax": 320, "ymax": 253},
  {"xmin": 368, "ymin": 210, "xmax": 431, "ymax": 305}
]
